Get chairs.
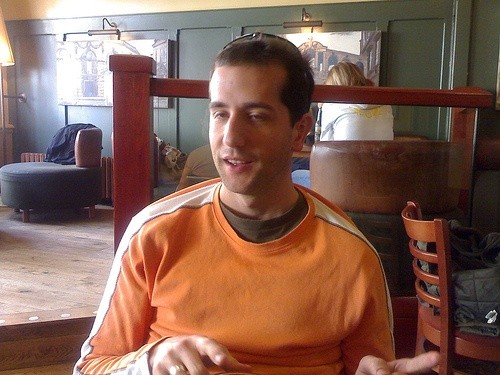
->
[401,200,500,375]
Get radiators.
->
[21,152,114,202]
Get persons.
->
[71,30,441,375]
[176,62,395,188]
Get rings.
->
[169,365,185,375]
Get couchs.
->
[0,127,103,223]
[309,139,451,290]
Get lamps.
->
[87,17,121,36]
[282,7,324,29]
[0,7,27,165]
